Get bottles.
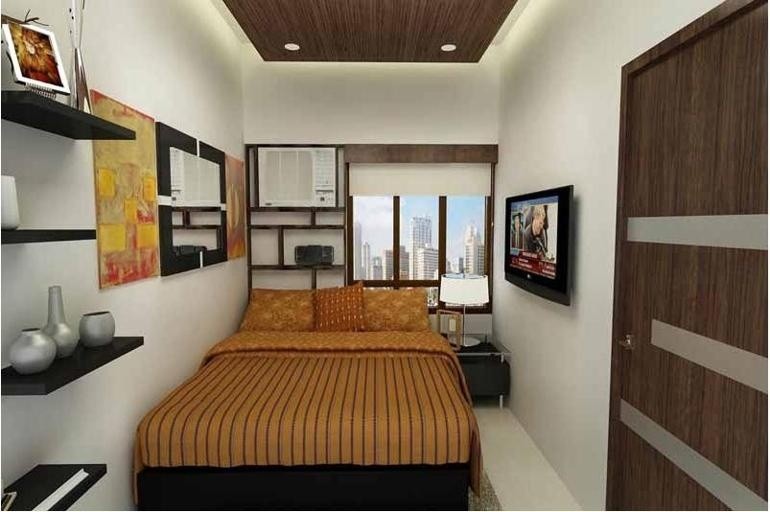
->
[9,287,115,375]
[2,174,20,228]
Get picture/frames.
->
[2,13,72,97]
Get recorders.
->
[294,244,335,265]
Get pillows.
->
[239,289,431,334]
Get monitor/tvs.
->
[503,185,574,308]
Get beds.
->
[132,287,484,511]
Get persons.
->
[511,204,547,261]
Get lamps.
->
[438,272,490,348]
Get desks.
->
[441,333,510,410]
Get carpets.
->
[469,467,502,512]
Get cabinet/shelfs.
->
[1,89,149,511]
[245,145,354,289]
